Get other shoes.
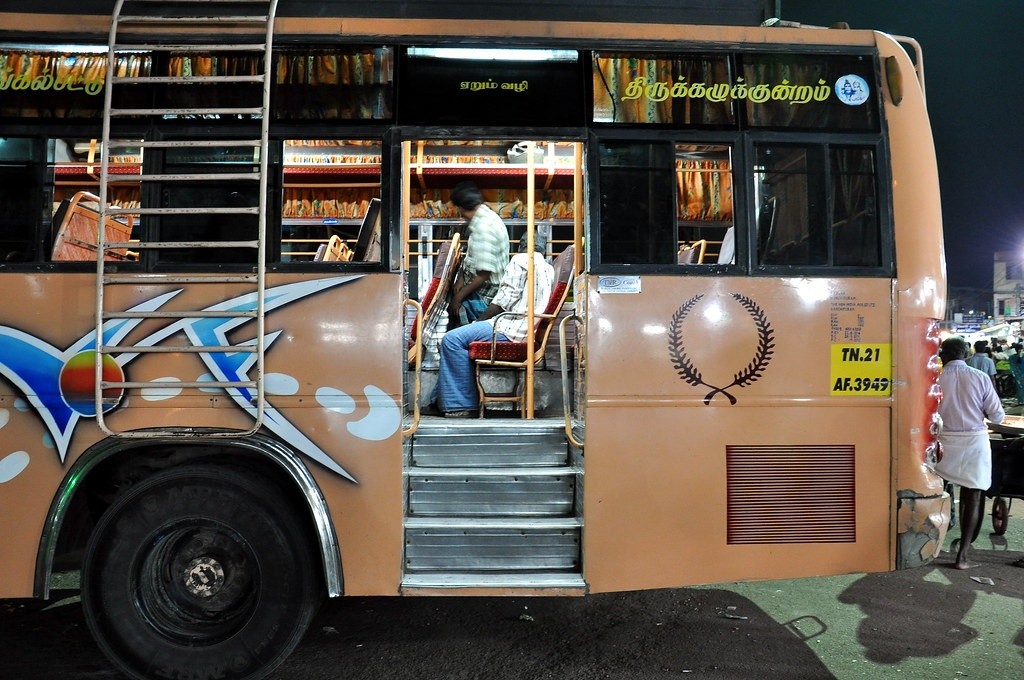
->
[445,405,487,417]
[421,404,443,415]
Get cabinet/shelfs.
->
[51,146,732,226]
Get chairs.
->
[406,233,461,365]
[678,239,707,264]
[314,197,383,262]
[52,189,134,263]
[467,243,577,419]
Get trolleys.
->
[988,435,1024,535]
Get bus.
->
[0,7,952,679]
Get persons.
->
[936,338,1005,571]
[961,329,1024,405]
[448,182,510,326]
[419,231,553,418]
